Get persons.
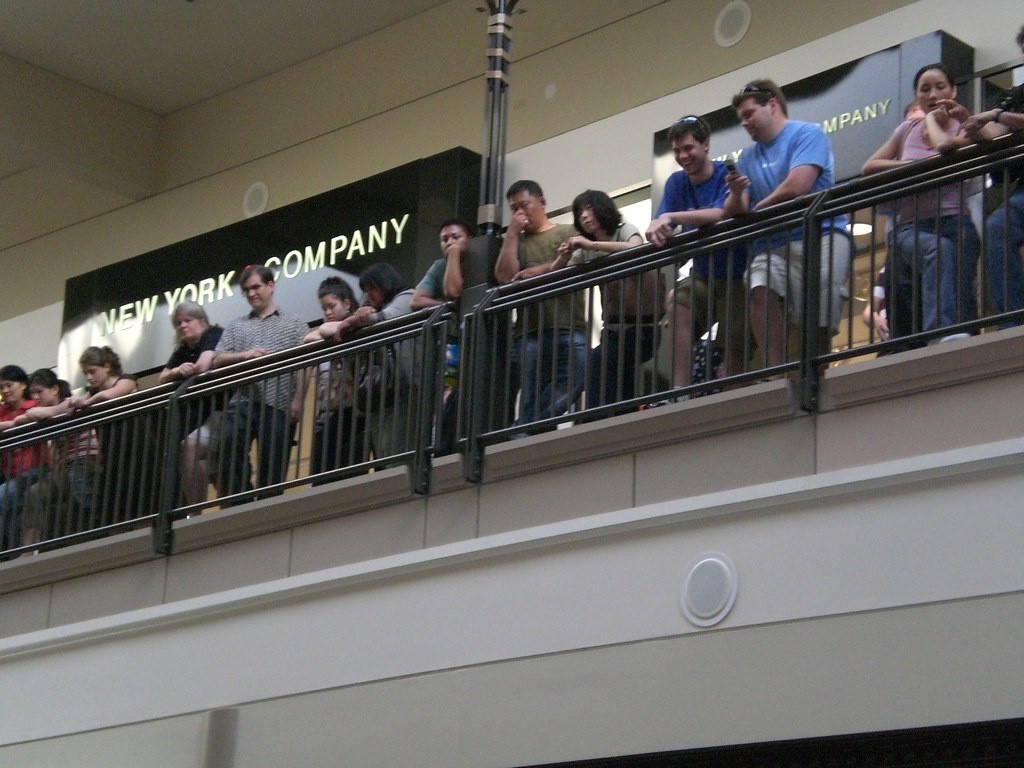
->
[336,263,415,472]
[159,301,225,518]
[550,190,663,424]
[66,346,138,538]
[410,219,491,459]
[862,24,1024,357]
[494,180,588,440]
[0,365,100,563]
[645,115,744,407]
[304,277,360,487]
[212,265,315,506]
[723,80,851,386]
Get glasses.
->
[673,117,706,130]
[740,86,775,96]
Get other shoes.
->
[926,332,973,346]
[640,395,689,410]
[507,424,527,438]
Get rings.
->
[965,123,975,131]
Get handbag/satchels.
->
[359,347,405,401]
[330,358,358,407]
[689,339,723,398]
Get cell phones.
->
[723,159,740,177]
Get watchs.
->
[995,107,1006,123]
